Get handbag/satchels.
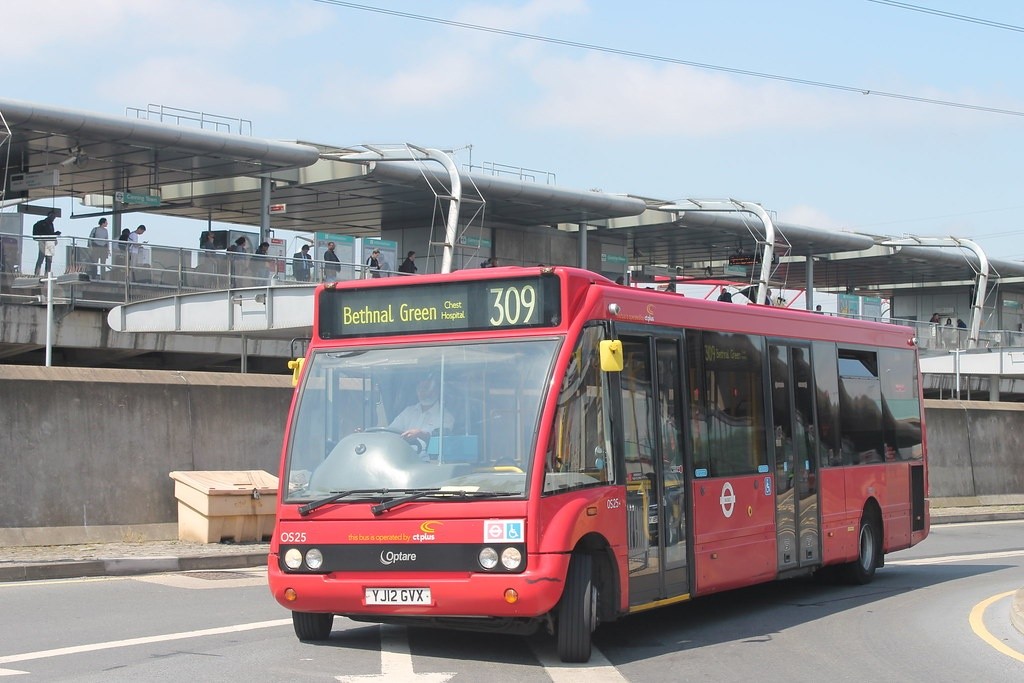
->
[249,258,257,273]
[87,226,97,247]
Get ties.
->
[303,254,307,269]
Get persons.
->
[324,242,341,280]
[383,377,453,465]
[366,250,383,278]
[35,211,61,276]
[718,288,731,302]
[255,242,268,277]
[930,313,940,325]
[488,258,498,267]
[957,319,968,348]
[666,284,675,292]
[128,225,148,282]
[765,288,774,306]
[816,305,821,311]
[616,276,624,285]
[398,251,417,276]
[234,237,246,260]
[944,319,953,347]
[206,232,222,266]
[293,244,313,282]
[88,218,111,279]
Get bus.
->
[267,265,930,662]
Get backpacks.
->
[33,220,44,238]
[118,232,134,250]
[398,260,406,271]
[226,245,237,255]
